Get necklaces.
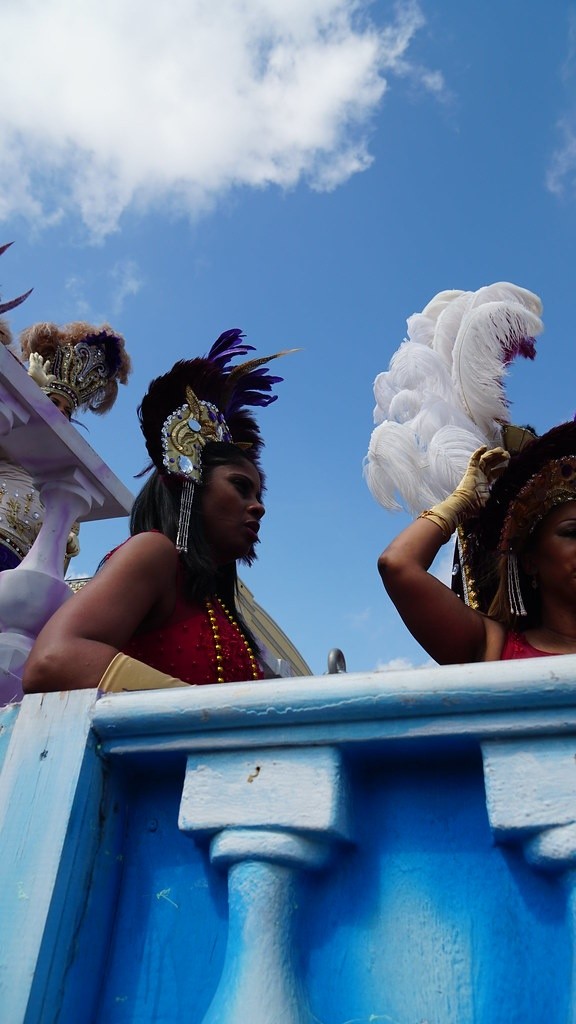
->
[204,592,259,684]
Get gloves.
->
[97,650,191,695]
[418,445,510,546]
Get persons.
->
[364,281,576,666]
[17,320,130,422]
[22,327,301,691]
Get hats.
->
[19,319,130,416]
[135,328,304,560]
[451,416,576,633]
